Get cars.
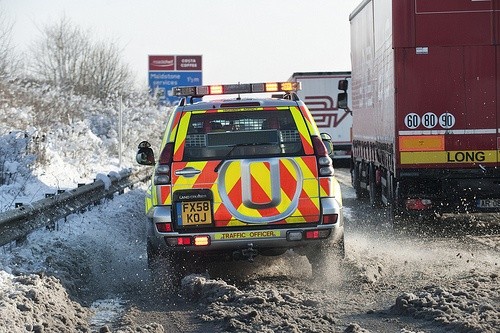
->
[137,82,346,273]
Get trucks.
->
[289,72,352,168]
[349,1,497,229]
